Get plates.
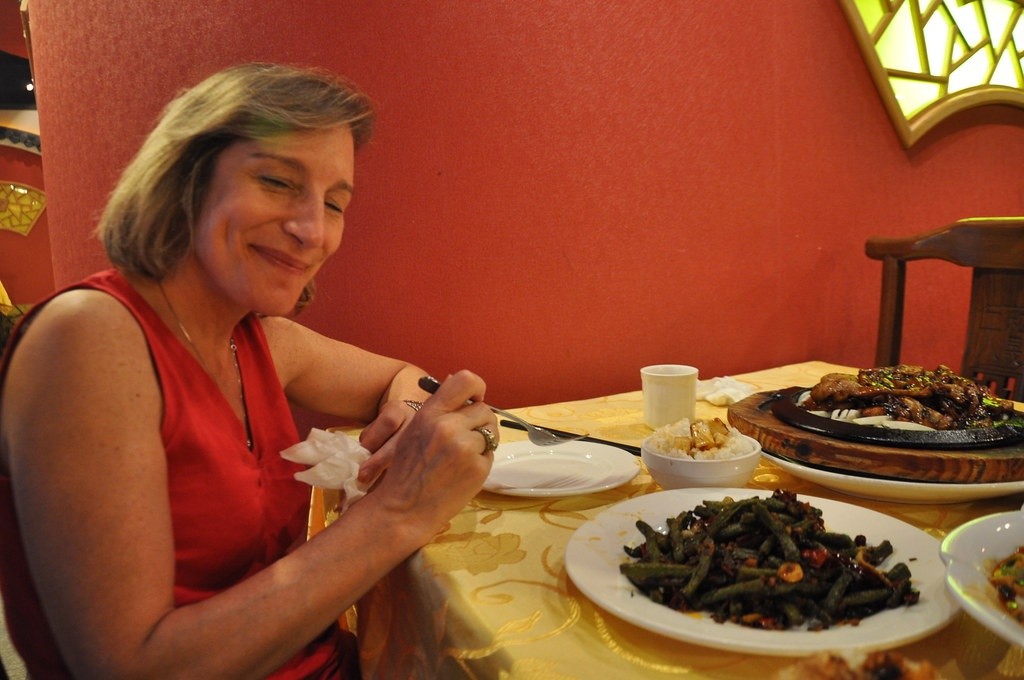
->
[563,485,964,657]
[758,449,1024,505]
[482,440,642,499]
[771,383,1024,450]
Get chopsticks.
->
[499,418,642,456]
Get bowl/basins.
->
[939,506,1024,651]
[641,434,763,491]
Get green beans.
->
[620,489,922,629]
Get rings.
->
[476,426,498,454]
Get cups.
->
[639,364,699,432]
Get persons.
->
[0,60,498,680]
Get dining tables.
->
[301,358,1024,680]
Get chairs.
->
[864,215,1024,403]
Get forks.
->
[418,375,591,448]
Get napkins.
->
[696,374,760,409]
[278,427,381,511]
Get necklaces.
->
[155,277,251,447]
